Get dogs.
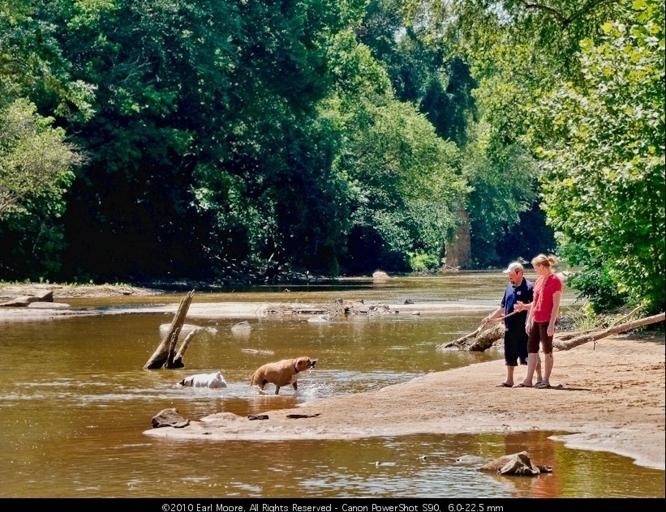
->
[250,356,317,395]
[179,370,227,388]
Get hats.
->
[502,262,524,275]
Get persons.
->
[480,262,545,387]
[512,253,562,389]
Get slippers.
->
[494,381,552,389]
